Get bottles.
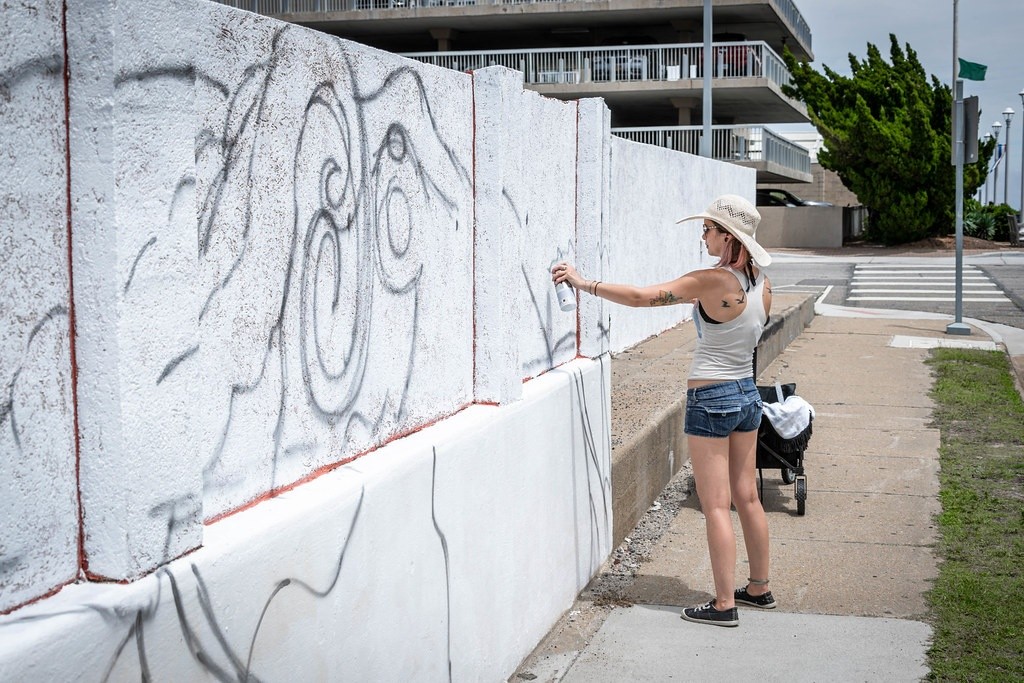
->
[553,266,577,312]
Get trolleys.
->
[754,384,807,514]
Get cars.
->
[757,188,835,207]
[1018,224,1024,243]
[592,50,667,77]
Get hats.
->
[677,194,772,267]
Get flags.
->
[990,144,1006,173]
[958,58,987,81]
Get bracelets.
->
[595,282,602,296]
[590,280,596,295]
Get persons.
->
[551,195,777,626]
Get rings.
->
[563,266,566,270]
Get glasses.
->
[702,224,720,233]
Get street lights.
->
[1020,91,1024,224]
[991,122,1002,202]
[984,133,991,204]
[1002,108,1015,204]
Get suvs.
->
[697,32,761,77]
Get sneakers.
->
[734,584,777,608]
[682,598,739,626]
